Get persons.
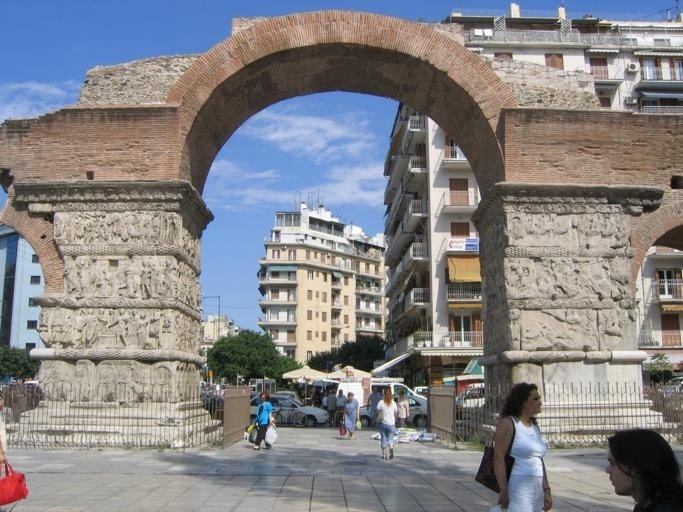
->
[309,386,347,427]
[366,386,383,427]
[490,383,553,512]
[342,393,360,441]
[0,400,7,465]
[396,390,410,428]
[605,428,683,512]
[251,392,275,450]
[373,387,398,460]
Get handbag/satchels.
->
[356,421,362,430]
[249,426,260,443]
[0,473,28,507]
[339,425,347,436]
[475,445,515,494]
[265,428,278,445]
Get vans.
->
[457,382,485,408]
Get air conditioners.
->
[626,62,639,73]
[625,96,637,105]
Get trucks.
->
[337,381,429,409]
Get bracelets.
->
[542,486,550,491]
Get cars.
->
[657,377,682,403]
[201,381,329,427]
[360,394,428,426]
[413,384,428,395]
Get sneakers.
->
[383,448,394,460]
[253,445,272,450]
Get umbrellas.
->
[327,365,372,393]
[282,365,327,401]
